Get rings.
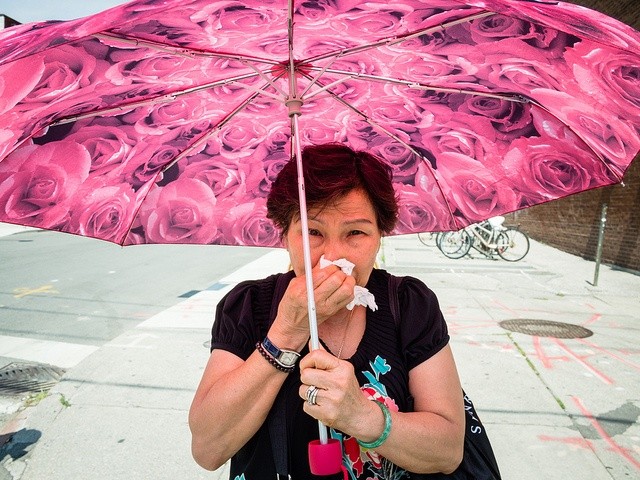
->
[305,385,320,405]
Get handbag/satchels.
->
[387,276,502,480]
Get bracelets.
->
[357,400,392,449]
[256,341,293,373]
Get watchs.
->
[263,337,301,368]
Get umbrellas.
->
[0,0,640,475]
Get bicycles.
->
[417,216,529,262]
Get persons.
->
[189,143,466,480]
